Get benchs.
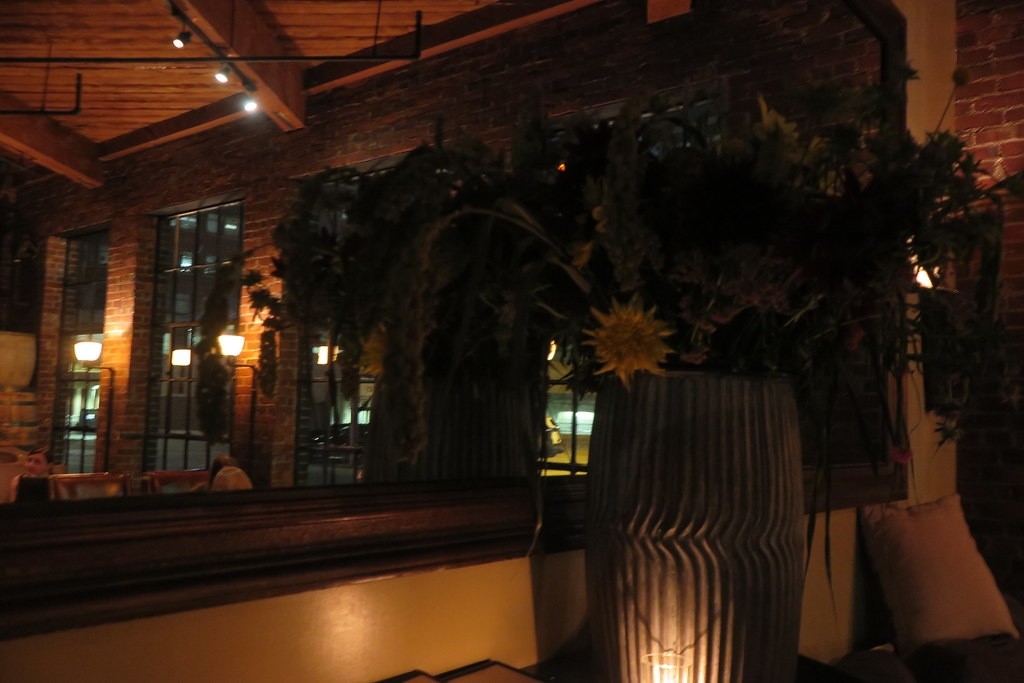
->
[142,468,215,495]
[12,472,133,500]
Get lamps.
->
[214,66,231,84]
[172,22,192,49]
[216,334,255,369]
[73,341,115,373]
[240,97,257,112]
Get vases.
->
[583,365,806,683]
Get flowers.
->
[235,62,1024,623]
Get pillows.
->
[855,493,1020,658]
